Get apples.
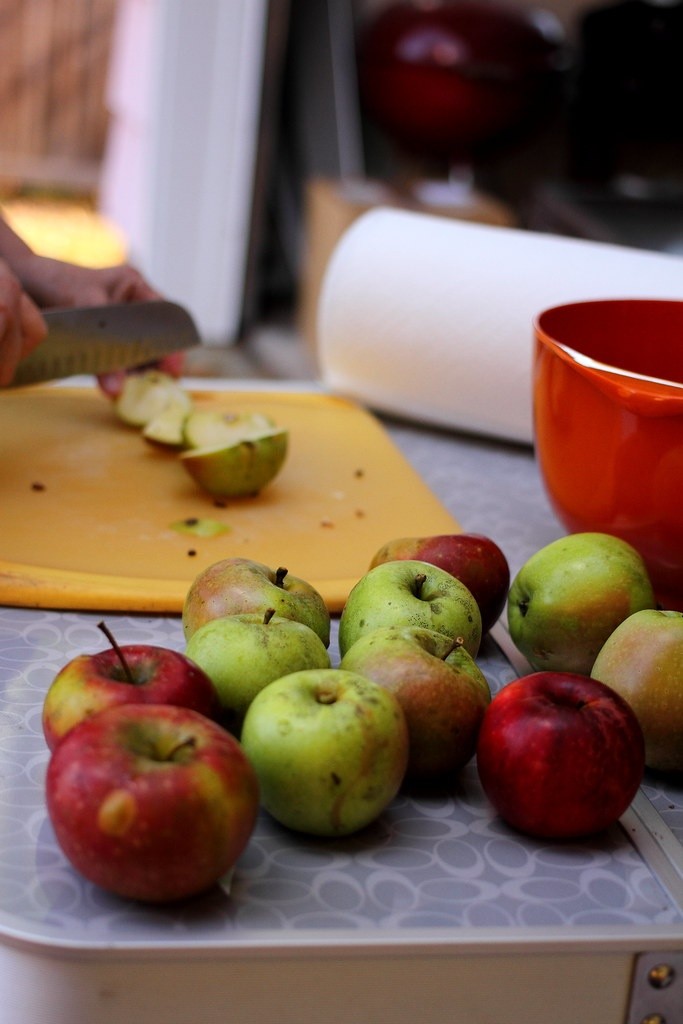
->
[40,531,683,904]
[113,369,287,495]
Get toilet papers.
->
[315,204,683,447]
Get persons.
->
[0,219,186,401]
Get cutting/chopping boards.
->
[0,377,464,618]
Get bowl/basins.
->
[535,299,683,584]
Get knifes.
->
[1,300,202,390]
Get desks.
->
[0,376,683,1024]
